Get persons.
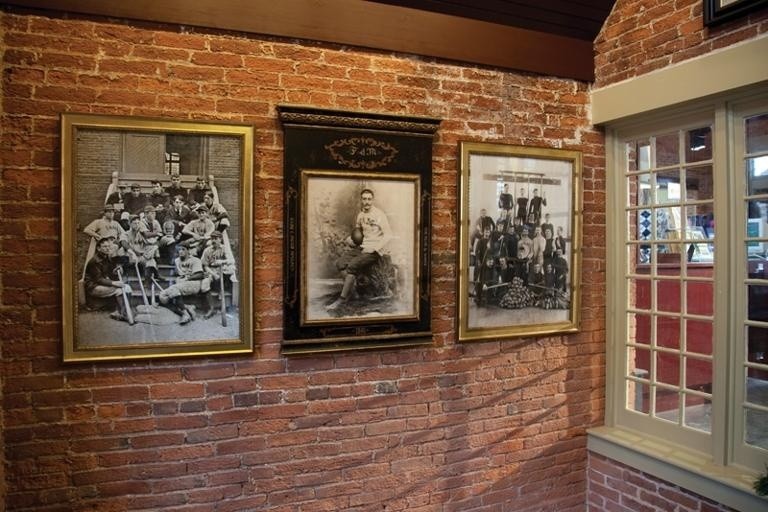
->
[83,174,230,326]
[326,189,392,310]
[470,184,569,308]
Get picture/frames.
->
[279,106,443,358]
[456,138,583,343]
[59,111,255,362]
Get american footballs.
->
[351,227,364,246]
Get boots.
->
[111,293,132,320]
[202,288,217,319]
[159,295,197,325]
[215,278,222,301]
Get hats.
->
[104,180,222,249]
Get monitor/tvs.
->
[747,218,768,257]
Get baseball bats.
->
[151,272,156,304]
[135,264,150,305]
[151,276,163,291]
[220,264,227,326]
[116,268,135,324]
